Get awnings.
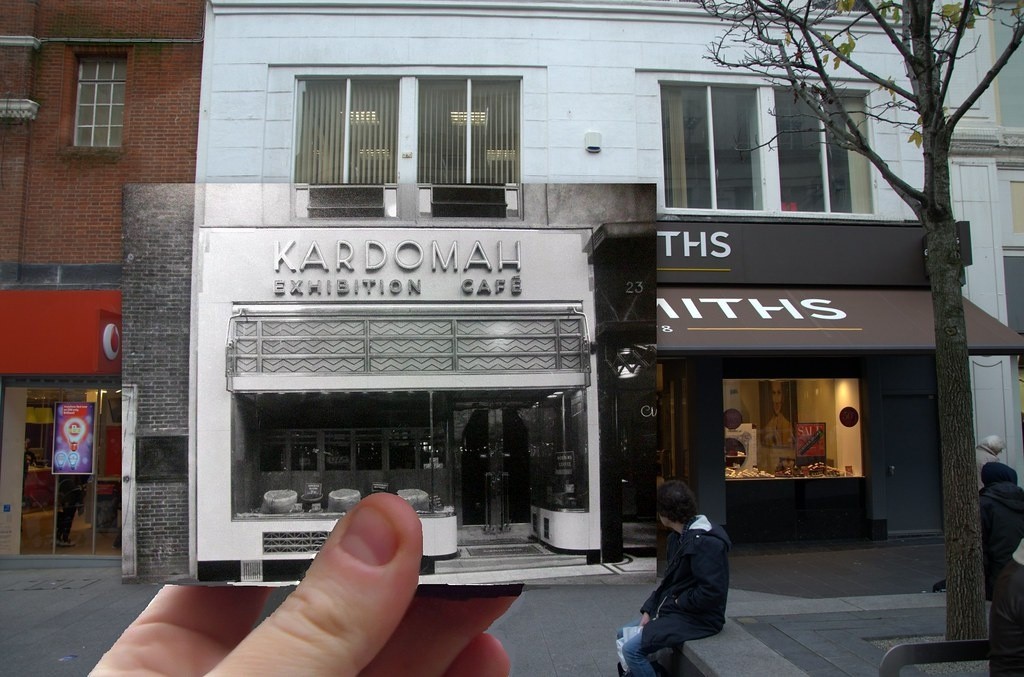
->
[225,304,593,395]
[656,288,1024,363]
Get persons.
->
[85,491,527,677]
[617,478,733,677]
[763,380,793,446]
[987,539,1024,677]
[54,475,91,548]
[977,462,1023,599]
[22,438,39,509]
[974,433,1006,489]
[112,484,122,550]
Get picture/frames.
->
[759,381,799,449]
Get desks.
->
[97,477,123,485]
[25,466,56,509]
